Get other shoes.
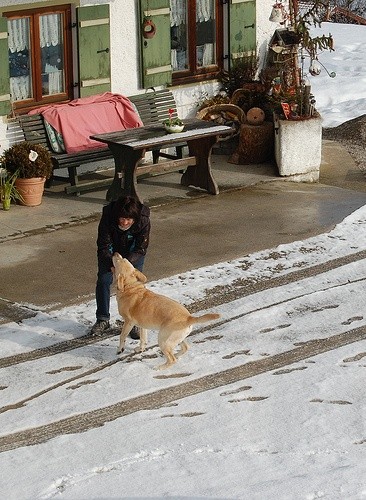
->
[91,319,110,337]
[130,326,141,339]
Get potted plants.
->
[201,44,277,165]
[3,141,54,207]
[0,167,26,210]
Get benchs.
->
[17,88,196,197]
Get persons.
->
[88,196,151,339]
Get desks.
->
[89,118,235,205]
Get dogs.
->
[111,252,222,371]
